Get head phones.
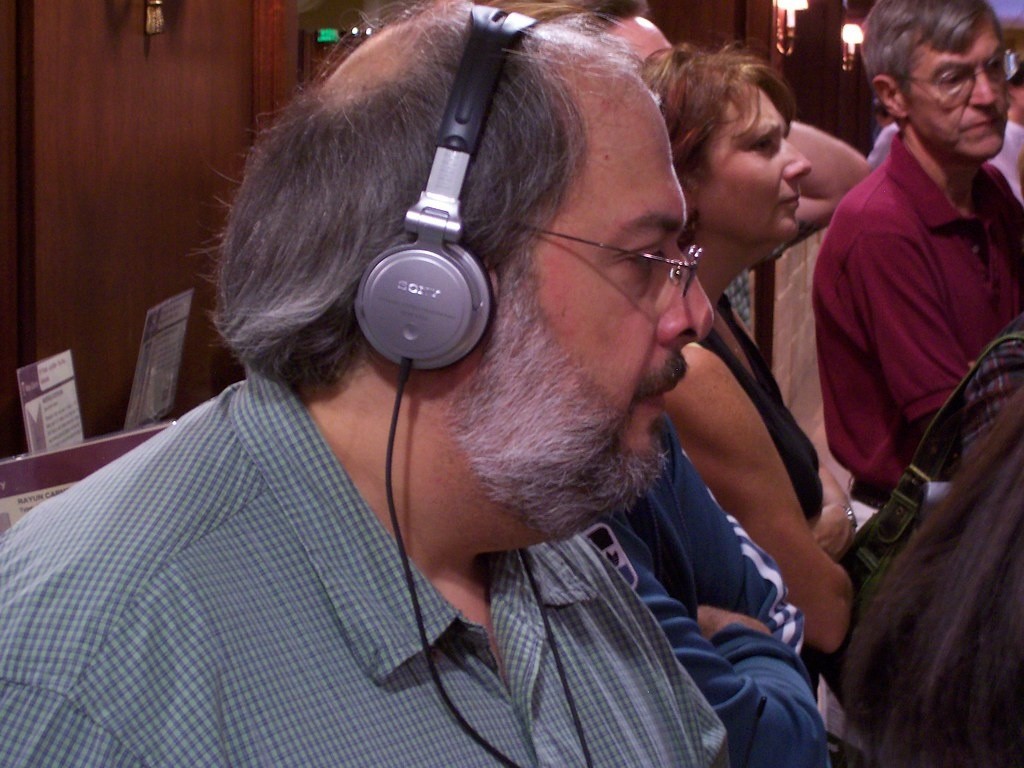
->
[352,3,539,371]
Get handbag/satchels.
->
[819,331,1024,709]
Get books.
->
[16,349,85,455]
[123,288,193,433]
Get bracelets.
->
[836,501,855,522]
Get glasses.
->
[902,48,1019,110]
[513,221,705,298]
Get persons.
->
[0,1,732,768]
[515,2,1024,768]
